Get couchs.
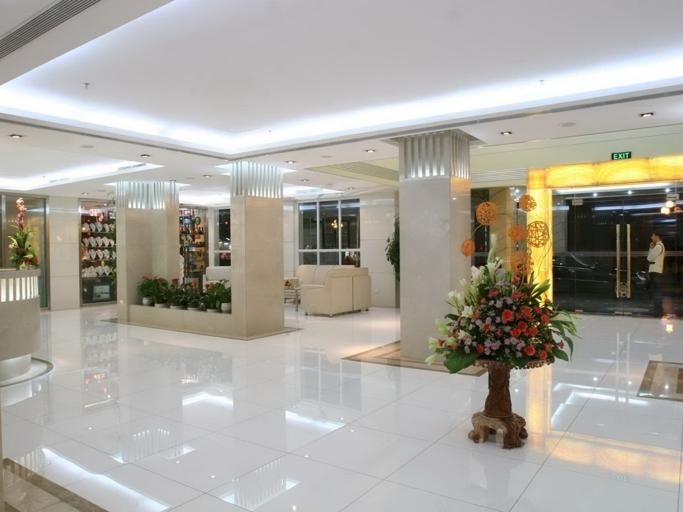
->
[295,264,371,318]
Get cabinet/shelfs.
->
[180,216,209,276]
[82,214,117,302]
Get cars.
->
[554,252,650,296]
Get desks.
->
[464,357,556,452]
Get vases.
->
[142,297,231,313]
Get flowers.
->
[425,255,579,377]
[137,275,231,306]
[7,196,40,269]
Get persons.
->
[644,228,665,318]
